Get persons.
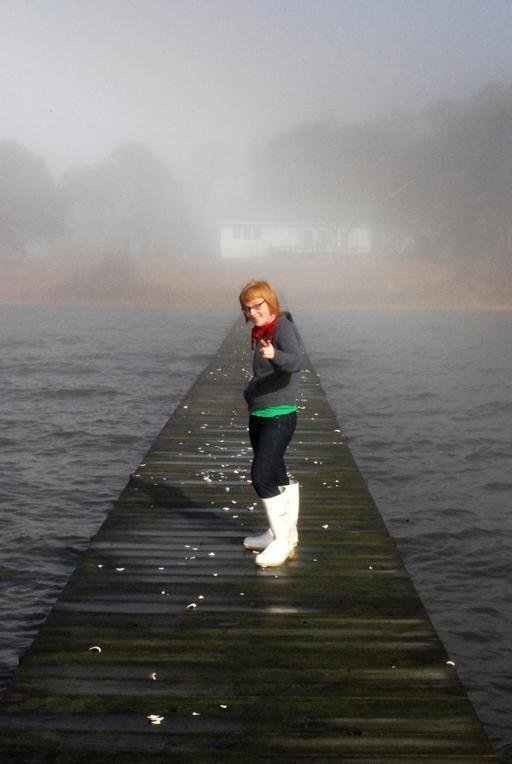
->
[238,280,307,570]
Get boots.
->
[255,487,296,567]
[243,479,300,551]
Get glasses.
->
[241,301,267,315]
